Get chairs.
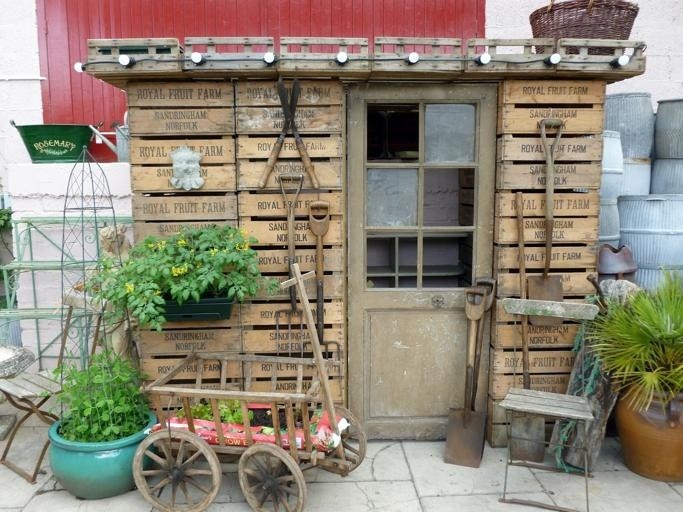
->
[498,298,602,512]
[0,293,107,484]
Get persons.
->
[86,222,137,376]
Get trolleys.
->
[131,261,366,512]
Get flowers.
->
[100,224,284,333]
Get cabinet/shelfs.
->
[367,232,465,288]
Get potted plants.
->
[39,350,157,499]
[584,269,683,482]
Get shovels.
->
[471,277,497,462]
[443,286,489,468]
[510,191,558,463]
[527,119,564,328]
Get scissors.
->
[257,75,321,190]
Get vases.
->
[155,295,234,323]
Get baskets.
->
[528,0,640,56]
